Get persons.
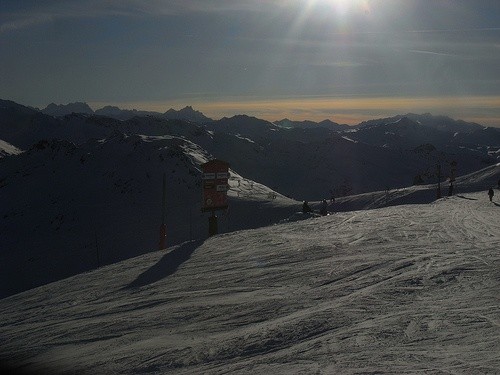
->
[303,200,313,214]
[159,223,166,249]
[487,187,495,201]
